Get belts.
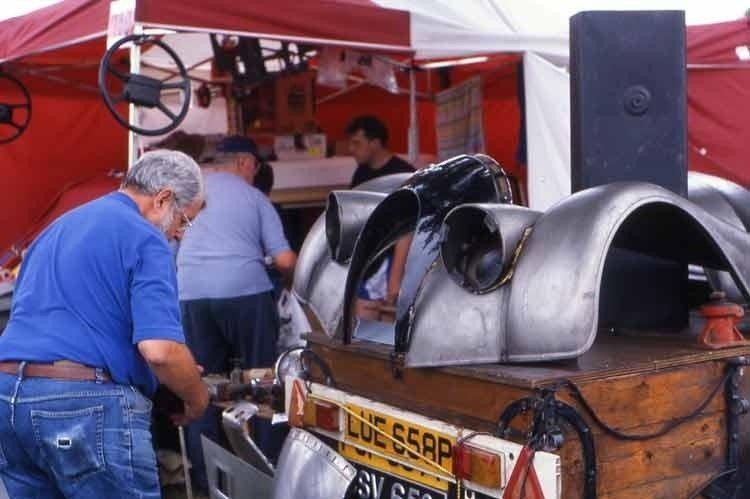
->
[0,361,109,382]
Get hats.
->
[216,135,256,152]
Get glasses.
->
[175,195,193,231]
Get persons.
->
[176,135,296,499]
[0,148,211,499]
[254,167,291,241]
[345,115,416,188]
